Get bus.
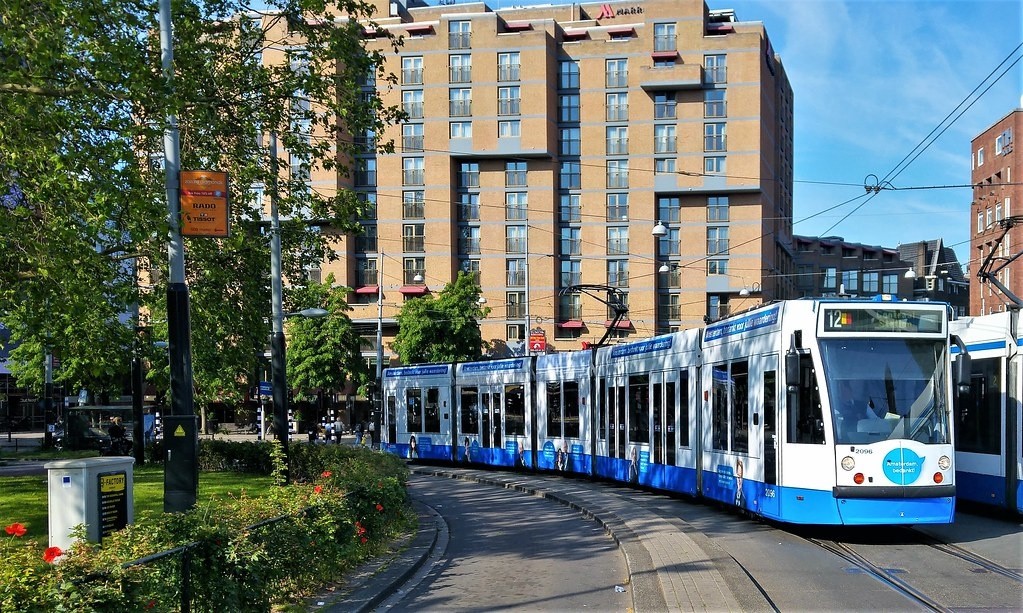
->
[382,283,973,527]
[67,405,155,447]
[944,215,1023,515]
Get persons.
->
[629,447,639,484]
[735,461,747,509]
[515,441,529,467]
[555,448,565,471]
[355,421,365,448]
[334,417,343,445]
[463,438,472,462]
[407,438,418,458]
[308,420,319,444]
[367,418,375,448]
[563,441,573,471]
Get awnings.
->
[405,24,433,34]
[651,51,678,59]
[399,286,427,293]
[607,28,635,37]
[705,26,733,31]
[604,320,631,329]
[504,23,531,29]
[562,320,585,328]
[354,286,379,294]
[365,29,377,34]
[563,31,588,37]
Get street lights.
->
[130,252,168,467]
[268,127,329,486]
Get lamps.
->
[659,262,670,273]
[651,222,669,237]
[478,297,488,303]
[905,268,916,280]
[412,273,424,282]
[739,288,751,298]
